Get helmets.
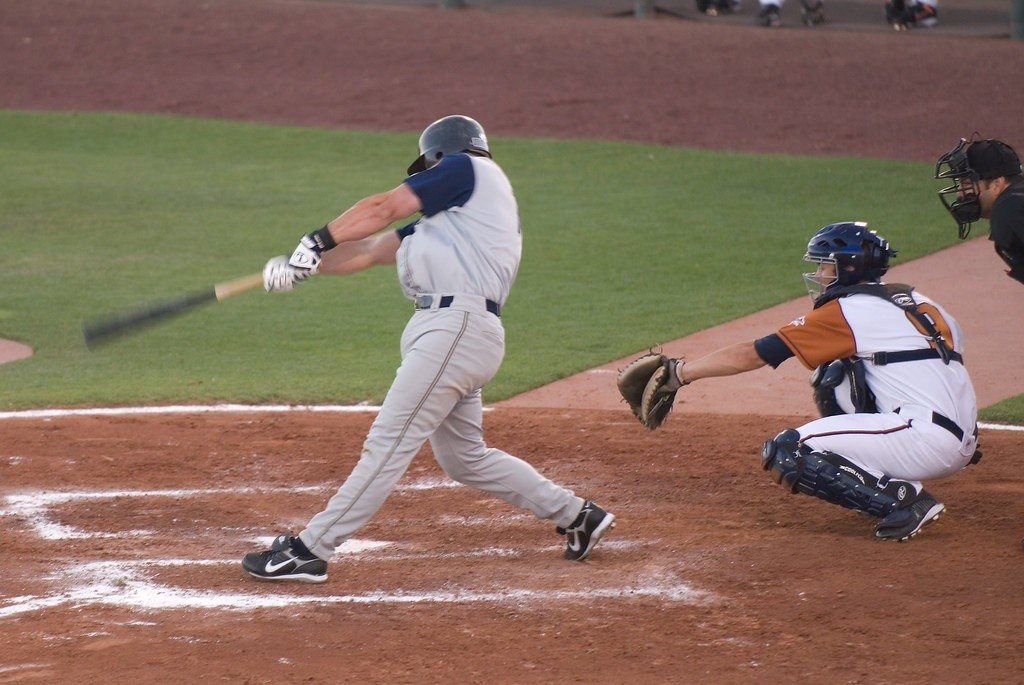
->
[948,138,1022,179]
[802,222,898,266]
[407,114,491,175]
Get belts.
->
[893,406,964,441]
[416,295,500,316]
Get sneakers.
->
[554,499,615,561]
[242,531,329,584]
[875,489,947,542]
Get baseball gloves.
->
[616,341,687,430]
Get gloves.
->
[263,254,322,293]
[288,223,337,291]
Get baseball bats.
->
[82,264,320,351]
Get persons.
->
[241,114,617,587]
[618,222,982,540]
[696,0,937,32]
[935,131,1024,288]
